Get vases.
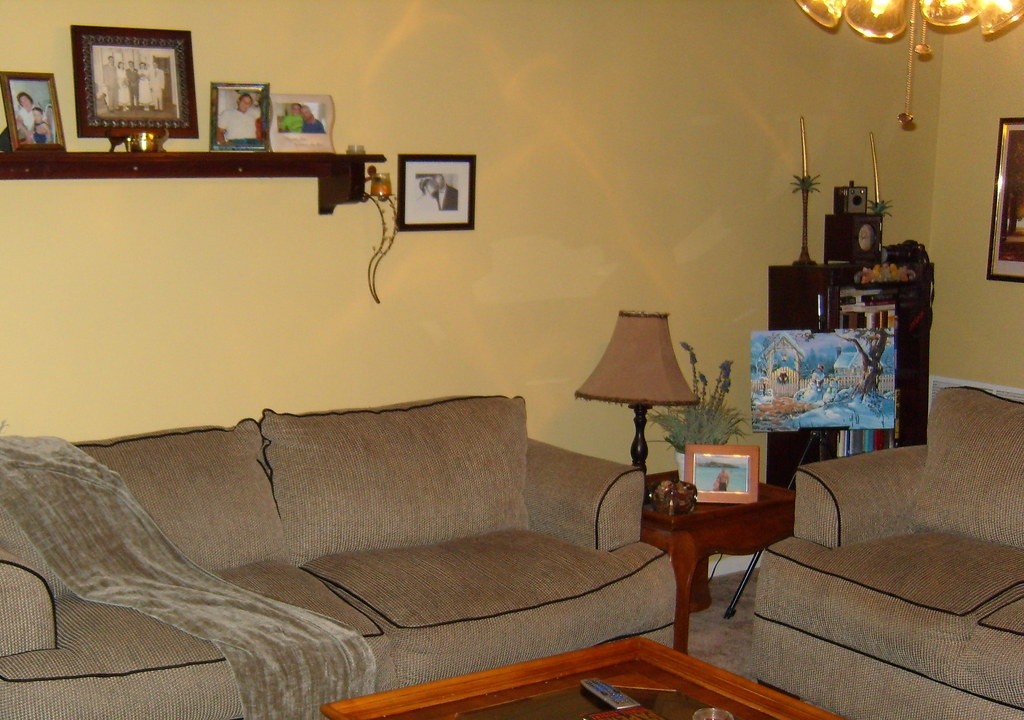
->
[674,452,687,481]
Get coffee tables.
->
[319,635,846,720]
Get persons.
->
[280,102,324,134]
[416,174,457,212]
[716,468,729,491]
[103,56,165,112]
[17,91,52,144]
[217,92,261,146]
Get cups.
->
[131,132,158,152]
[371,173,391,196]
[692,708,734,720]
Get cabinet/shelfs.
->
[765,265,938,487]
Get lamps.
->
[578,311,698,477]
[794,1,1021,132]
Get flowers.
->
[649,343,743,453]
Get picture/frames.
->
[270,95,336,151]
[69,25,200,135]
[1,72,67,149]
[206,83,270,151]
[396,153,478,230]
[987,117,1024,283]
[687,445,762,505]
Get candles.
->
[798,114,808,177]
[869,131,883,204]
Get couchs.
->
[1,397,675,720]
[751,387,1023,719]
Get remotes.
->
[580,676,641,710]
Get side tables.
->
[635,471,802,661]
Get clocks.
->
[825,213,883,264]
